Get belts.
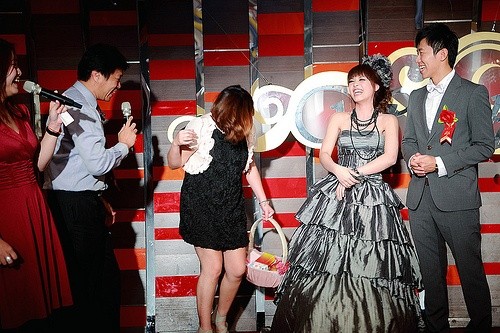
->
[425,178,429,186]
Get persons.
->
[400,23,494,333]
[167,85,275,333]
[38,44,138,333]
[269,52,428,333]
[0,35,75,333]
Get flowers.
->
[276,262,291,275]
[437,104,458,144]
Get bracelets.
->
[260,200,269,205]
[47,128,63,137]
[434,163,438,172]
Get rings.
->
[5,257,11,263]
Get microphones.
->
[22,81,83,109]
[121,102,133,152]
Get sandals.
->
[198,327,213,333]
[211,304,230,333]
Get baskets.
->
[245,217,288,288]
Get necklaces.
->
[350,107,380,161]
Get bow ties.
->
[426,82,445,93]
